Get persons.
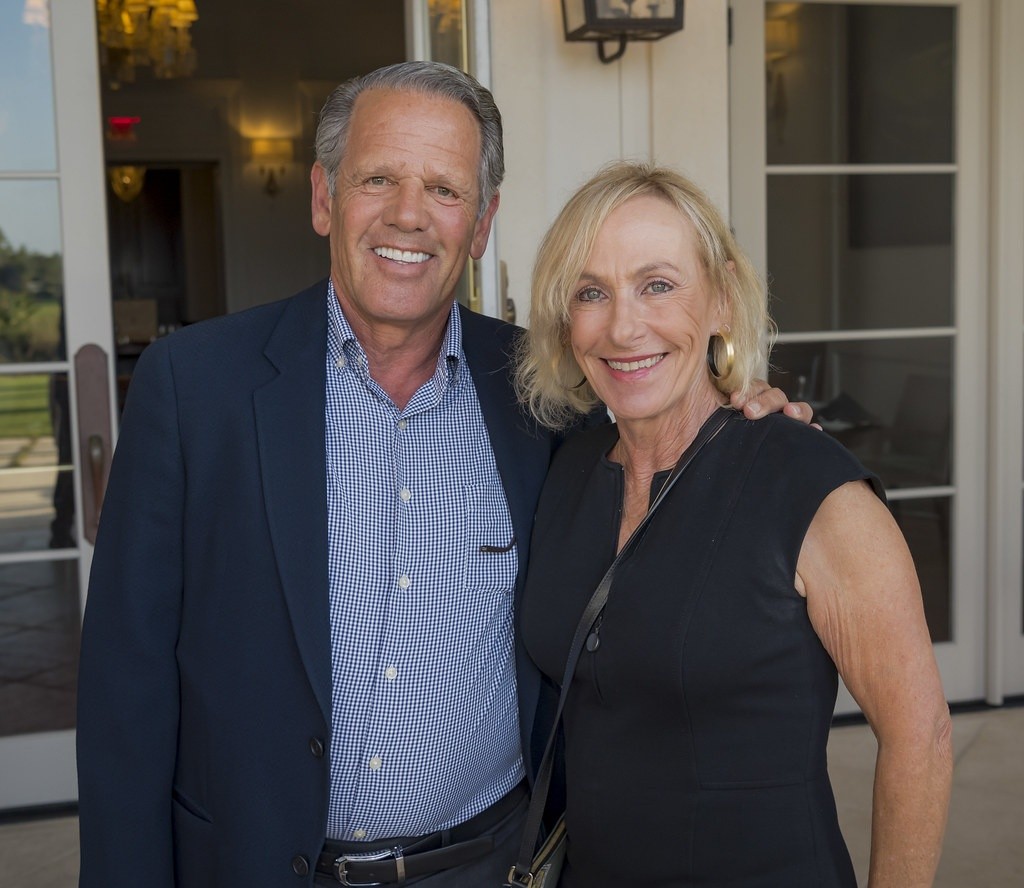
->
[77,60,823,888]
[512,163,955,888]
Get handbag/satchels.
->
[501,812,569,888]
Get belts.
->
[316,776,530,887]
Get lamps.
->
[562,0,684,63]
